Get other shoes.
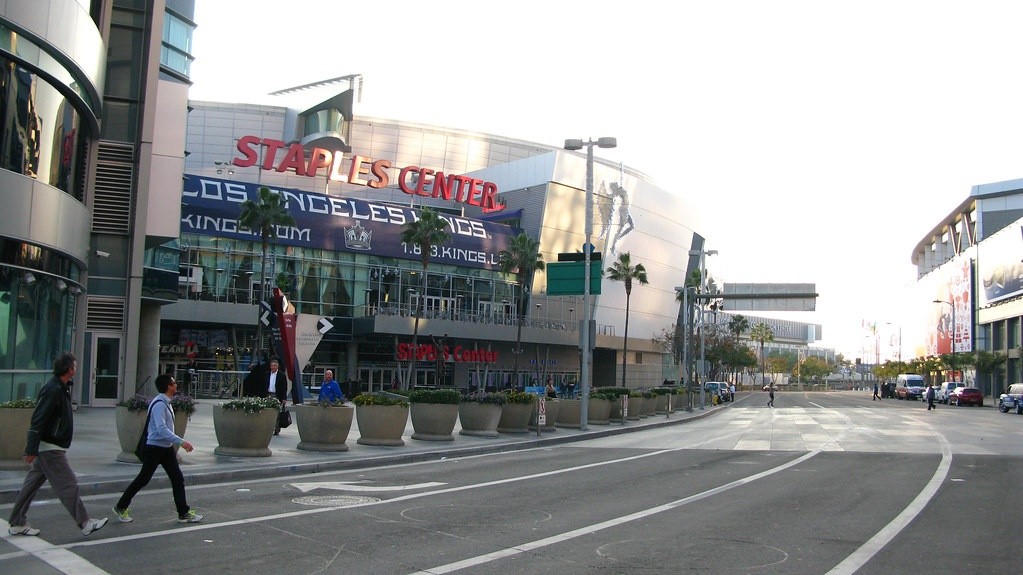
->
[273,432,278,435]
[771,405,774,407]
[767,403,769,406]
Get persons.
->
[392,376,400,390]
[767,382,774,407]
[926,384,936,410]
[8,352,108,536]
[112,374,204,523]
[713,382,735,406]
[545,377,575,398]
[260,359,288,435]
[318,370,344,405]
[873,384,881,401]
[185,359,255,395]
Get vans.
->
[894,372,927,400]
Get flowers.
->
[116,392,199,422]
[218,396,282,416]
[0,397,38,408]
[505,393,537,404]
[409,388,461,404]
[351,393,408,407]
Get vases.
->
[496,404,534,434]
[357,404,409,446]
[212,404,279,457]
[116,405,189,464]
[554,399,581,428]
[0,408,37,470]
[410,402,459,440]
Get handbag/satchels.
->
[134,432,148,461]
[280,403,292,427]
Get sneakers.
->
[81,517,108,535]
[113,505,134,522]
[177,508,204,523]
[9,525,40,536]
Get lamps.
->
[0,291,11,305]
[56,277,67,292]
[70,284,82,297]
[19,272,36,286]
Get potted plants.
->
[597,387,630,422]
[527,397,559,432]
[296,395,354,451]
[639,391,653,418]
[460,391,507,436]
[647,393,658,416]
[670,387,710,411]
[627,390,644,420]
[580,393,616,424]
[653,388,670,414]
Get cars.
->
[937,382,965,404]
[949,387,983,407]
[763,384,778,391]
[705,381,731,402]
[921,385,941,402]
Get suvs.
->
[999,382,1023,415]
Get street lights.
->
[564,135,619,433]
[761,337,766,387]
[675,249,720,414]
[866,335,880,366]
[933,300,959,383]
[795,345,803,387]
[886,322,902,374]
